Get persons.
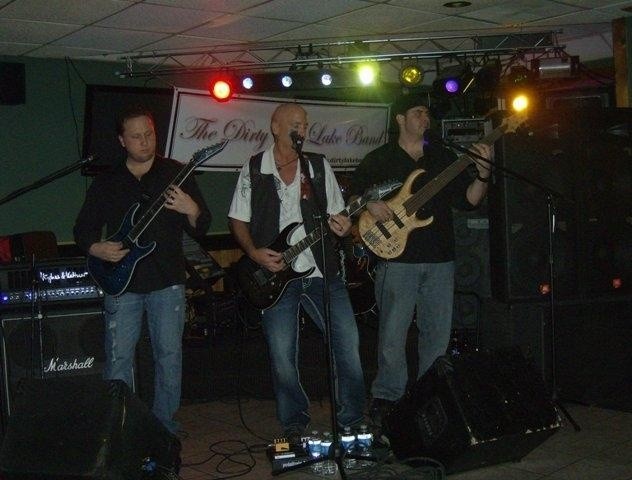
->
[227,102,368,444]
[346,94,493,427]
[73,102,211,435]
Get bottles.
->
[308,424,374,477]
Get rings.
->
[339,227,343,231]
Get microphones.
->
[423,129,449,147]
[289,131,305,154]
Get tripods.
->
[451,144,581,432]
[271,149,392,480]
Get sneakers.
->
[337,420,369,433]
[284,423,305,437]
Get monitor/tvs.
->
[81,84,204,176]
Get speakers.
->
[0,62,26,105]
[487,133,632,302]
[0,378,181,480]
[383,349,565,475]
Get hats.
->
[390,95,427,115]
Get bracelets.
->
[476,173,493,183]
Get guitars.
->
[86,139,228,298]
[227,178,403,313]
[358,108,527,259]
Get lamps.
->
[211,69,234,103]
[432,58,469,98]
[359,57,381,82]
[317,63,335,89]
[512,86,530,112]
[398,58,425,88]
[240,73,257,92]
[281,65,298,89]
